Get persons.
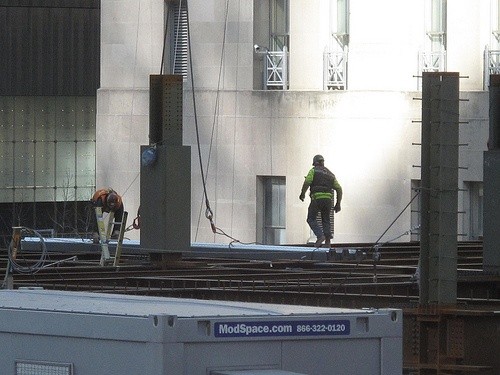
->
[90,188,124,243]
[300,155,342,248]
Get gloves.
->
[300,193,305,201]
[335,203,340,213]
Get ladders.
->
[98,210,128,266]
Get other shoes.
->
[114,230,120,240]
[322,243,332,248]
[315,236,324,246]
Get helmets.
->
[106,193,117,208]
[312,154,325,165]
